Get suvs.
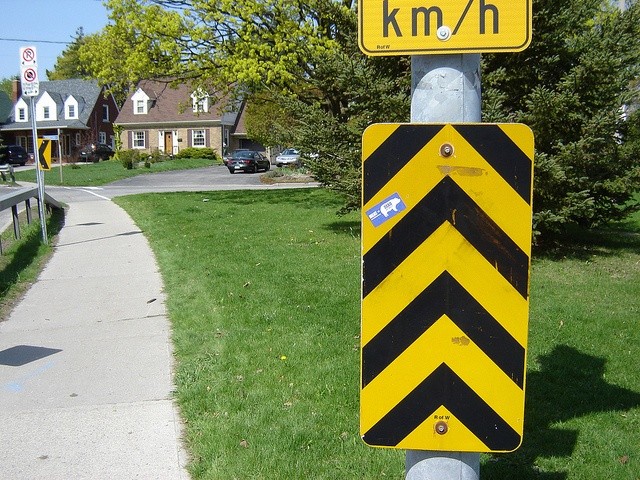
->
[80,143,115,161]
[0,145,29,166]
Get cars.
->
[276,148,300,167]
[226,150,270,174]
[222,148,250,165]
